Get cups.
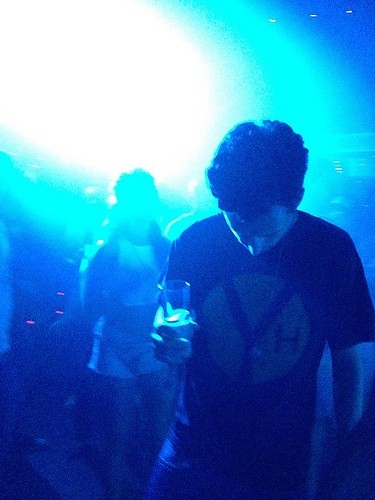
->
[160,279,192,337]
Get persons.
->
[79,169,190,500]
[142,120,374,500]
